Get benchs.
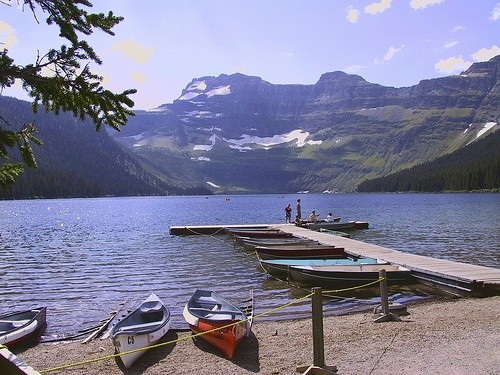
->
[120,321,163,331]
[193,301,221,305]
[190,308,242,320]
[0,319,30,324]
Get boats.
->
[0,306,48,360]
[296,190,309,194]
[291,216,341,224]
[111,292,172,370]
[224,227,412,291]
[182,288,252,361]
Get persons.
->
[284,203,292,223]
[327,213,333,220]
[296,198,301,219]
[309,210,319,223]
[294,215,302,226]
